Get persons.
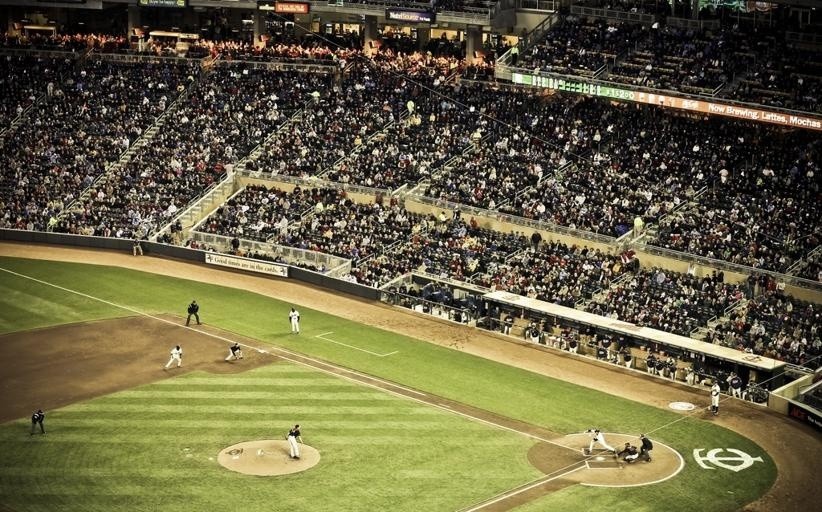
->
[613,442,639,462]
[639,434,653,457]
[30,409,47,435]
[165,345,182,368]
[289,308,300,334]
[0,0,393,288]
[585,429,615,453]
[285,424,303,459]
[710,379,720,415]
[225,343,243,360]
[379,0,822,402]
[185,301,202,326]
